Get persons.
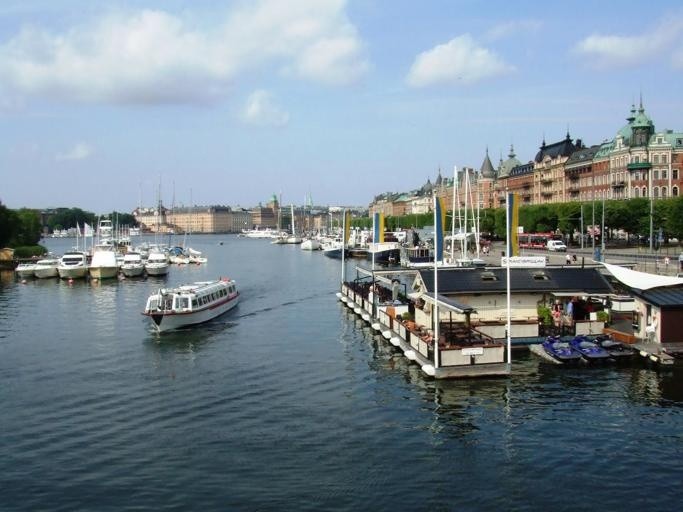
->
[573,253,577,263]
[678,252,683,270]
[486,246,490,256]
[483,245,486,254]
[566,253,570,264]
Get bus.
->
[515,232,563,250]
[477,230,491,248]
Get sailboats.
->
[272,188,314,244]
[52,173,209,267]
[436,162,487,267]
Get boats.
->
[593,330,637,362]
[541,333,582,365]
[138,275,241,335]
[10,251,171,282]
[568,332,612,363]
[237,224,288,240]
[301,227,442,270]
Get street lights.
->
[647,213,668,255]
[327,212,332,231]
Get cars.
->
[546,229,647,252]
